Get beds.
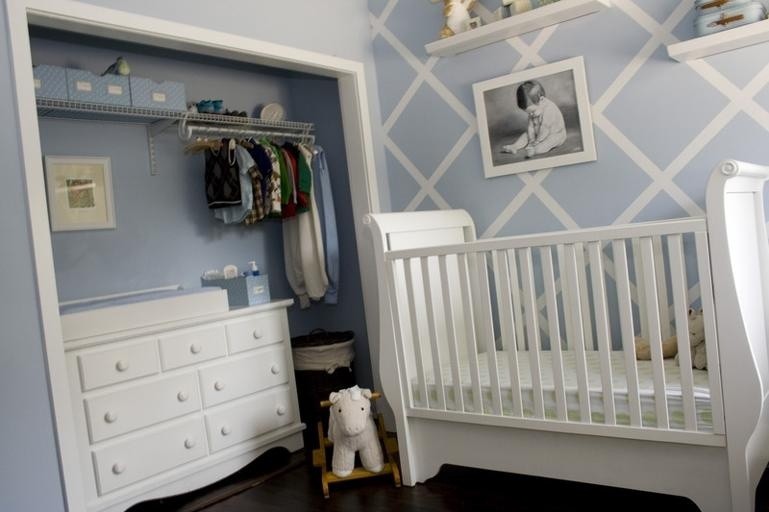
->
[360,159,768,512]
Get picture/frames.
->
[44,154,119,232]
[470,55,598,179]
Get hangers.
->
[179,130,317,155]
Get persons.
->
[499,82,570,156]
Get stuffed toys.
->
[675,307,708,370]
[436,0,479,39]
[312,386,401,495]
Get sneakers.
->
[196,99,224,114]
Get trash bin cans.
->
[290,328,357,437]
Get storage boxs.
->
[32,63,187,124]
